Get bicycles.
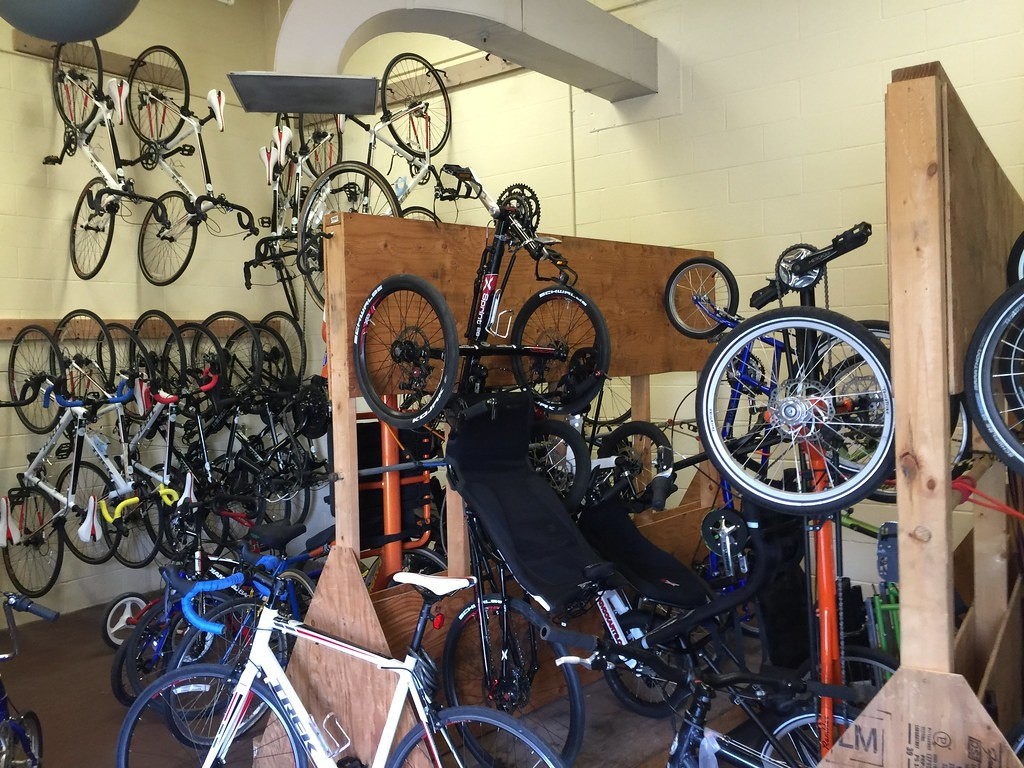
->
[0,38,1024,768]
